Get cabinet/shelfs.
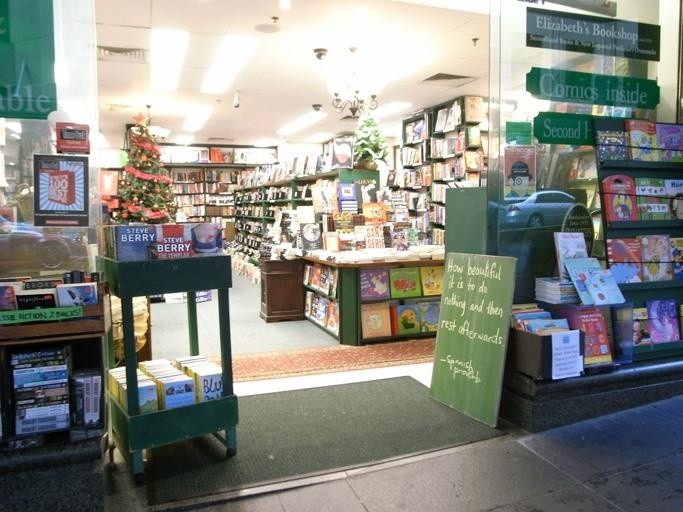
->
[302,247,447,347]
[402,94,516,246]
[163,166,380,346]
[94,250,239,485]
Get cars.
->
[499,190,578,229]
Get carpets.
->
[145,377,508,509]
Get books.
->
[2,120,107,440]
[161,145,231,239]
[504,116,682,379]
[98,223,223,302]
[109,355,223,414]
[231,96,501,340]
[101,169,126,221]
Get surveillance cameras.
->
[312,104,322,111]
[312,49,328,60]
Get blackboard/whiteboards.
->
[431,252,516,428]
